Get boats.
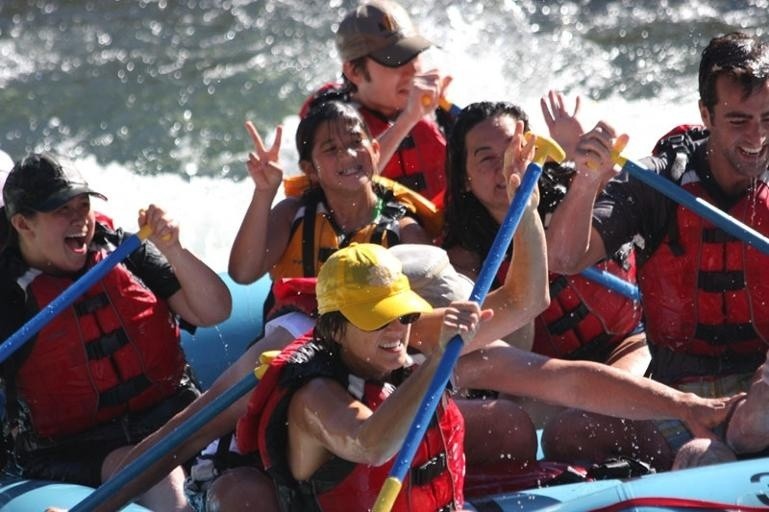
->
[0,262,767,511]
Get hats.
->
[331,0,441,69]
[1,151,109,222]
[385,242,477,311]
[315,241,435,333]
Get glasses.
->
[369,311,422,331]
